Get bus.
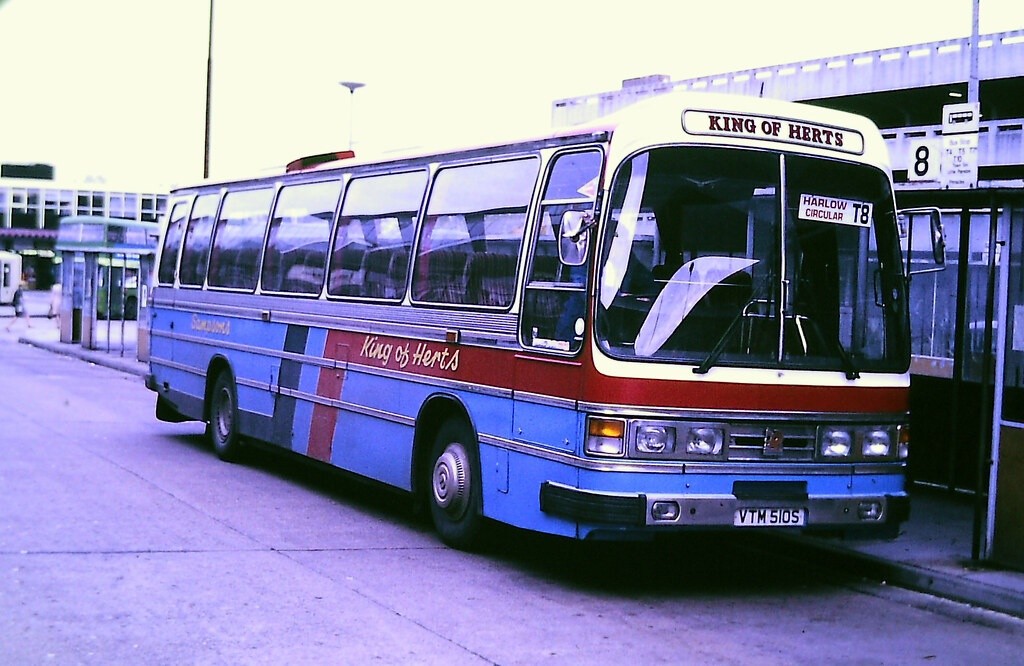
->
[144,92,948,551]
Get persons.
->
[50,280,63,329]
[6,281,33,333]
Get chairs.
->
[196,240,564,338]
